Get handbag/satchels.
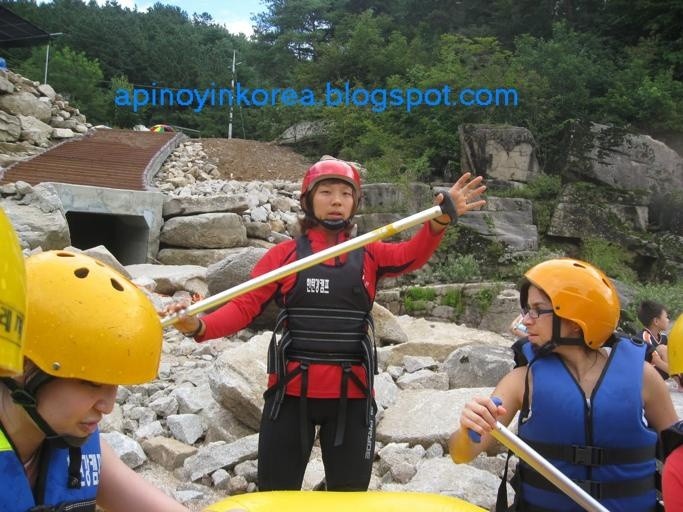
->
[183,320,202,338]
[432,218,449,226]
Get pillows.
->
[0,204,27,379]
[666,313,683,378]
[524,257,621,350]
[23,249,163,385]
[300,160,366,202]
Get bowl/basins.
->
[24,451,37,470]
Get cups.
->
[520,302,553,319]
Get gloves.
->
[201,489,492,512]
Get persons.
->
[0,250,197,512]
[447,257,681,510]
[633,301,683,393]
[156,160,487,490]
